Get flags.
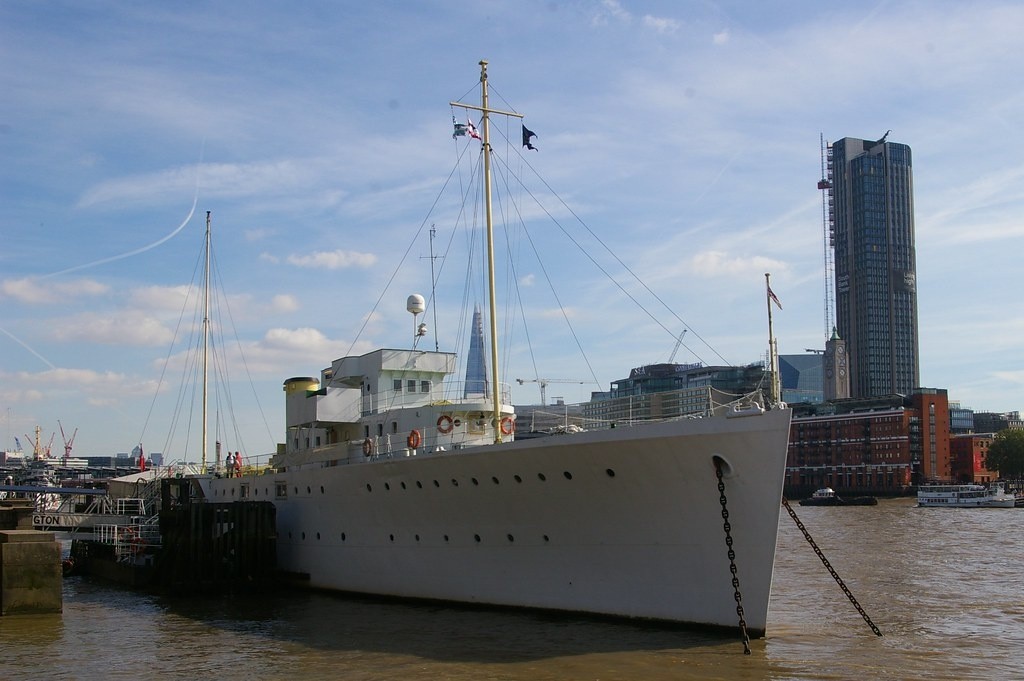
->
[453,115,482,140]
[767,285,782,309]
[139,446,146,471]
[520,123,542,153]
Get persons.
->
[226,452,242,478]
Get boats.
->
[20,460,61,511]
[184,56,792,636]
[916,485,1015,509]
[801,487,877,504]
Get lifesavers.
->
[167,466,173,477]
[501,416,516,434]
[437,415,454,434]
[131,538,145,552]
[363,437,373,458]
[408,429,421,449]
